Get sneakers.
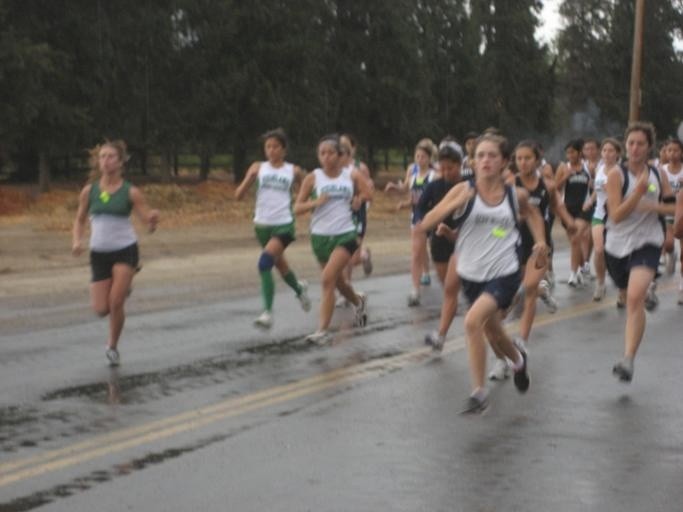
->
[424,334,529,415]
[104,345,119,367]
[419,275,430,285]
[659,251,683,305]
[642,280,659,312]
[254,248,371,345]
[408,291,420,307]
[611,360,634,382]
[513,262,625,318]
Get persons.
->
[72,139,160,365]
[236,126,311,328]
